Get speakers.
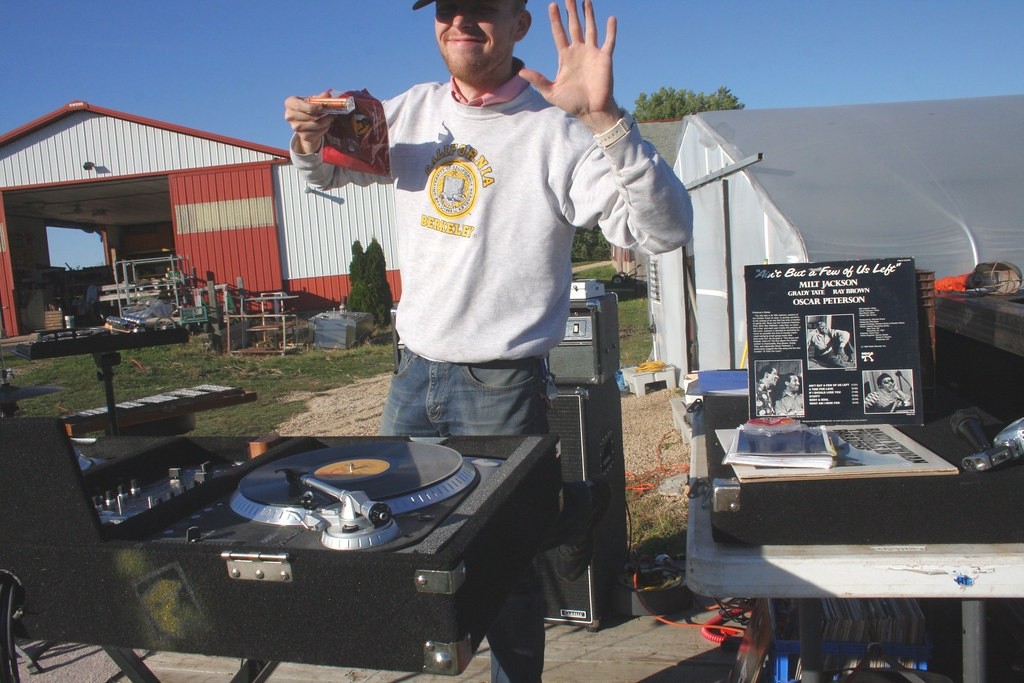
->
[529,377,629,632]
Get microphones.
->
[950,408,992,454]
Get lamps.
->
[83,162,94,170]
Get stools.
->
[621,366,676,396]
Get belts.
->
[463,351,546,370]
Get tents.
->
[612,101,1024,384]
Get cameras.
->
[963,419,1024,472]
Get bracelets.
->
[592,107,633,148]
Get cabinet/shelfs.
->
[114,254,374,356]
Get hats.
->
[412,0,528,10]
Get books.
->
[722,423,838,468]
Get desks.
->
[685,404,1024,683]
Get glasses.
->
[883,379,894,384]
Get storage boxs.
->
[918,271,938,392]
[765,594,932,683]
[570,278,604,300]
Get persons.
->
[755,365,804,415]
[808,321,855,368]
[864,373,911,413]
[284,1,692,683]
